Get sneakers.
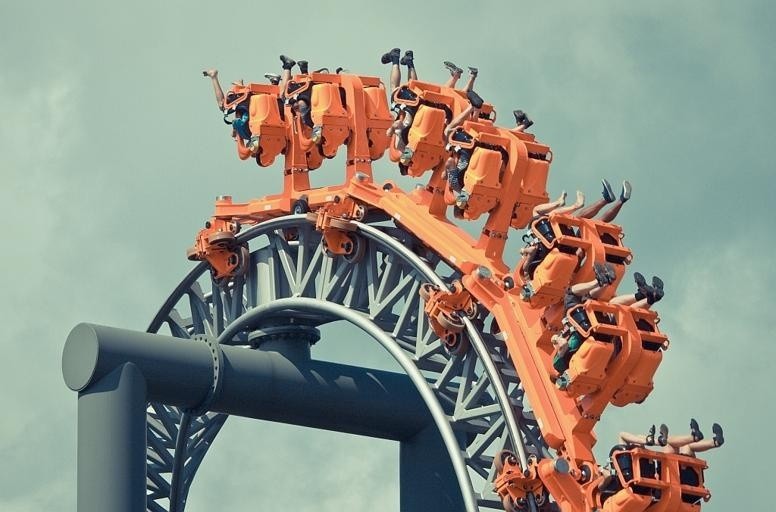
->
[199,69,219,78]
[523,112,534,130]
[400,50,414,67]
[312,68,329,74]
[230,79,244,87]
[601,177,617,203]
[620,179,632,203]
[279,55,296,69]
[262,72,282,84]
[513,109,526,122]
[467,65,480,77]
[651,275,665,301]
[465,88,485,108]
[335,66,343,75]
[381,47,402,65]
[297,59,308,75]
[633,271,654,298]
[443,60,464,80]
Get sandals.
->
[646,423,656,447]
[592,261,617,287]
[658,422,669,447]
[690,417,703,443]
[712,422,725,448]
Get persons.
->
[200,48,725,509]
